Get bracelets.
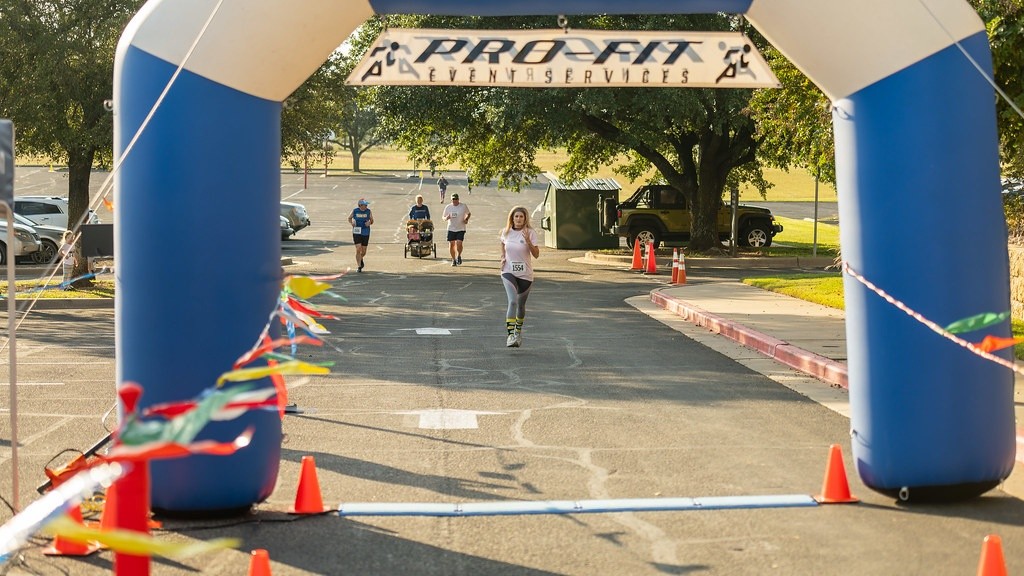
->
[466,218,468,220]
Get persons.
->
[89,208,98,280]
[348,199,374,272]
[500,206,540,347]
[409,195,430,221]
[442,194,471,266]
[58,231,76,291]
[431,168,435,177]
[466,168,473,195]
[438,175,449,204]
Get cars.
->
[0,220,43,266]
[11,212,70,264]
[278,201,311,239]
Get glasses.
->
[452,198,457,200]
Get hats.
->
[451,194,459,200]
[358,199,370,205]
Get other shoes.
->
[361,260,364,268]
[451,259,456,266]
[357,267,361,273]
[457,256,462,265]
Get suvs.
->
[602,184,784,251]
[12,195,101,231]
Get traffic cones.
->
[44,481,102,558]
[814,443,860,503]
[640,240,649,273]
[671,249,693,286]
[247,548,273,576]
[628,238,643,271]
[645,242,661,274]
[667,247,679,284]
[976,535,1009,576]
[287,455,338,516]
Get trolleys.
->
[403,218,437,262]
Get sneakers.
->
[506,333,518,346]
[514,333,522,346]
